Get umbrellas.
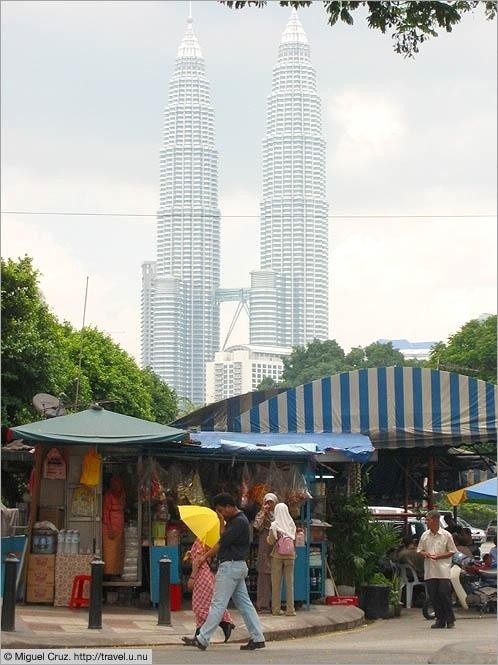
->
[175,504,221,548]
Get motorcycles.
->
[422,550,497,619]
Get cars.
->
[361,506,497,547]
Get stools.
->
[69,575,92,609]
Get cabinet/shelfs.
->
[309,541,326,598]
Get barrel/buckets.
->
[361,584,389,618]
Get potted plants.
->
[325,493,402,620]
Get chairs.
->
[398,564,429,610]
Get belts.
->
[221,558,244,563]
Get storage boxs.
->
[26,553,55,604]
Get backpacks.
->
[276,534,295,556]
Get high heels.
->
[223,622,235,643]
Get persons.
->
[405,512,497,569]
[266,503,298,617]
[181,531,235,646]
[253,491,279,614]
[385,513,497,617]
[181,494,265,652]
[415,509,456,629]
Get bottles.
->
[311,570,322,591]
[32,529,54,553]
[58,529,80,555]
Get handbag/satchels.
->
[206,554,220,575]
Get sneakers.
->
[240,639,267,651]
[258,608,271,614]
[447,623,456,628]
[182,636,207,651]
[431,621,446,628]
[286,611,296,616]
[272,609,285,616]
